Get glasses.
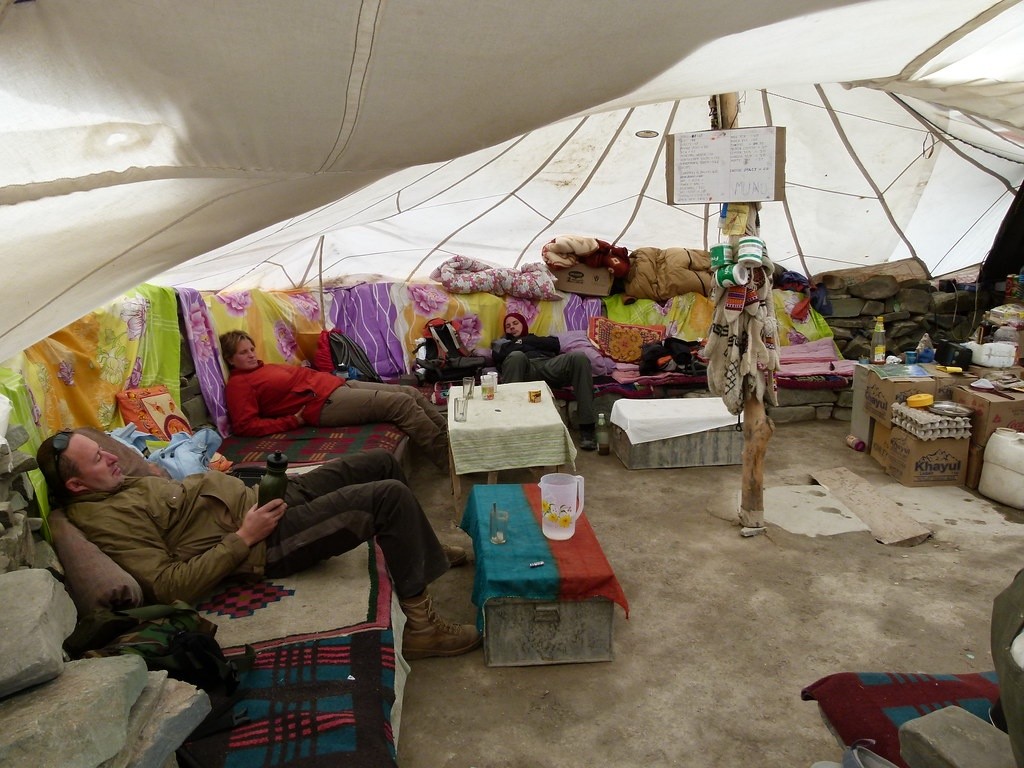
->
[52,431,73,483]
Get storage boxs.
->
[852,358,1024,489]
[550,260,614,297]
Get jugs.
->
[538,472,585,540]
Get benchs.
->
[1,265,852,768]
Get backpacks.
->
[425,318,485,370]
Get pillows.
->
[116,384,193,443]
[588,316,665,360]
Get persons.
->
[219,330,450,476]
[37,433,480,661]
[491,313,598,449]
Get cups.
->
[858,357,869,364]
[490,510,508,544]
[454,398,468,423]
[463,377,475,400]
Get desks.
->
[612,400,752,470]
[461,483,629,669]
[447,380,579,499]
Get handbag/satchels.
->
[173,632,239,697]
[329,332,381,381]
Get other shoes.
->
[843,739,900,768]
[579,424,597,449]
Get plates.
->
[927,401,975,416]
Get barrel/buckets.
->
[993,325,1021,366]
[978,427,1024,510]
[981,343,1015,368]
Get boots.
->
[402,588,482,660]
[441,543,465,566]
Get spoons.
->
[467,376,474,396]
[493,502,503,541]
[460,401,465,419]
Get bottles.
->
[869,317,886,365]
[906,351,917,365]
[335,363,349,381]
[487,372,498,393]
[258,449,289,509]
[480,375,494,400]
[917,346,935,363]
[845,434,865,452]
[596,413,610,456]
